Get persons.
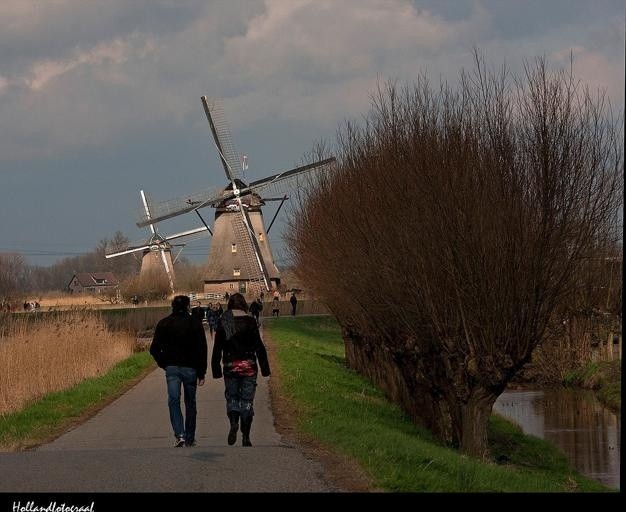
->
[271,296,281,316]
[149,295,208,447]
[191,301,224,341]
[290,293,297,316]
[23,300,41,313]
[131,294,138,308]
[211,293,270,446]
[248,298,263,329]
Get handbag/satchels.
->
[223,354,257,377]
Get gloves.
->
[264,376,270,381]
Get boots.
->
[240,414,253,447]
[227,410,240,445]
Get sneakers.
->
[173,435,199,448]
